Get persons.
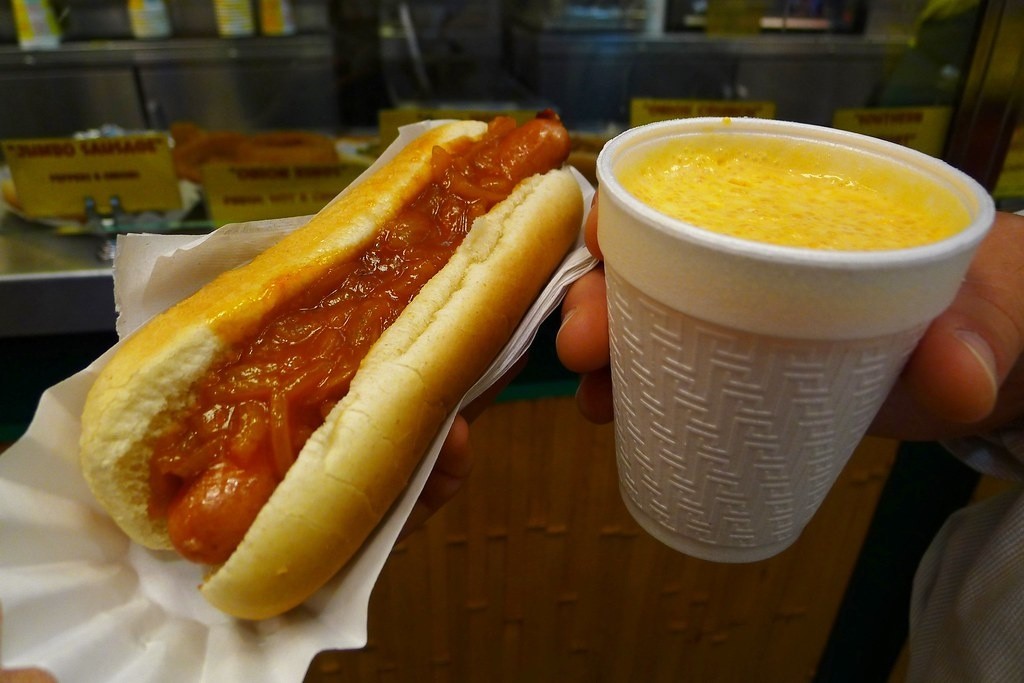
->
[0,184,1024,683]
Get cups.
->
[594,116,996,563]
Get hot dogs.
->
[77,109,583,620]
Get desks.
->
[0,394,907,683]
